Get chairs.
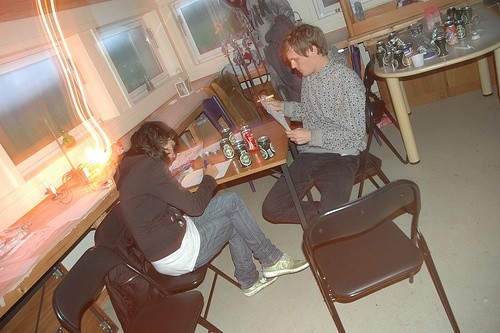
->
[93,202,242,333]
[302,179,462,333]
[52,245,224,333]
[203,44,397,199]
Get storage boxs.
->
[175,79,195,98]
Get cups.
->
[412,53,424,68]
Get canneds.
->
[433,6,472,58]
[240,125,258,153]
[257,136,276,161]
[376,32,414,70]
[220,138,235,159]
[232,139,253,168]
[222,128,235,144]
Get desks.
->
[373,9,500,166]
[171,117,309,229]
[0,51,218,333]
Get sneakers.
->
[242,271,279,296]
[263,254,311,278]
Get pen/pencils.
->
[204,161,207,169]
[256,94,275,103]
